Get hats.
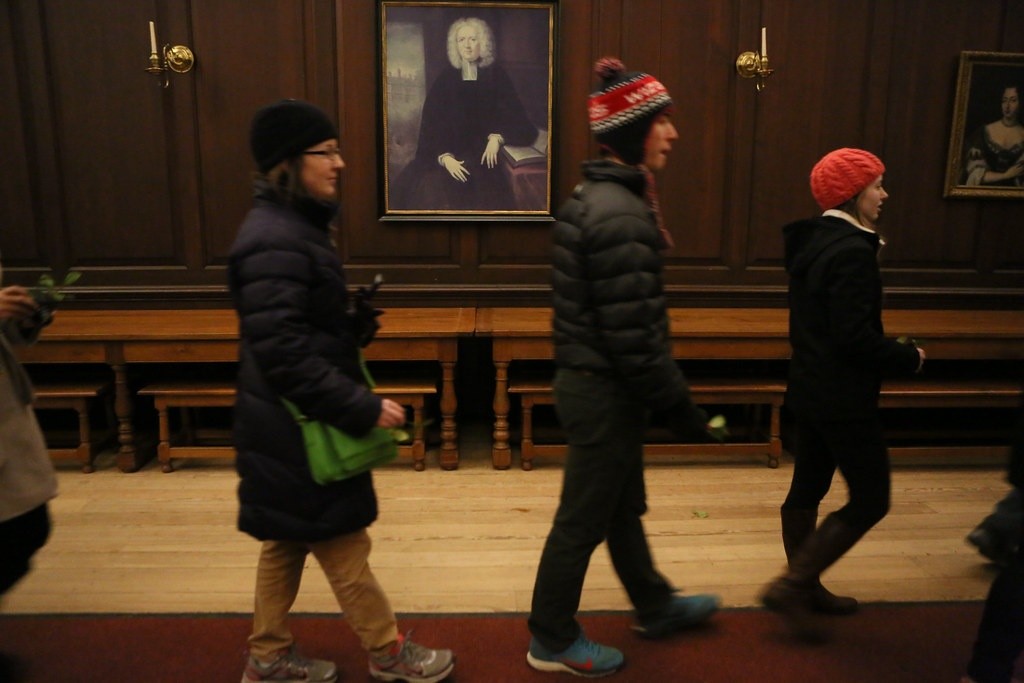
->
[250,98,338,175]
[811,147,886,210]
[587,57,672,165]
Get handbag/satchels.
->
[302,405,399,485]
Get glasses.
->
[300,147,341,161]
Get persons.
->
[961,433,1024,683]
[526,57,721,676]
[755,148,926,646]
[0,286,61,595]
[226,102,456,683]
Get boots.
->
[781,504,859,615]
[759,513,861,647]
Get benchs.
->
[503,378,786,466]
[134,376,439,475]
[877,378,1023,409]
[31,378,117,472]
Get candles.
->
[761,27,766,58]
[149,20,156,52]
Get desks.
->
[474,309,1024,468]
[25,304,474,468]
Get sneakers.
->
[635,590,721,639]
[365,633,458,683]
[527,623,623,678]
[240,647,338,683]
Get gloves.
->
[351,304,385,351]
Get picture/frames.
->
[377,1,559,223]
[941,46,1024,197]
